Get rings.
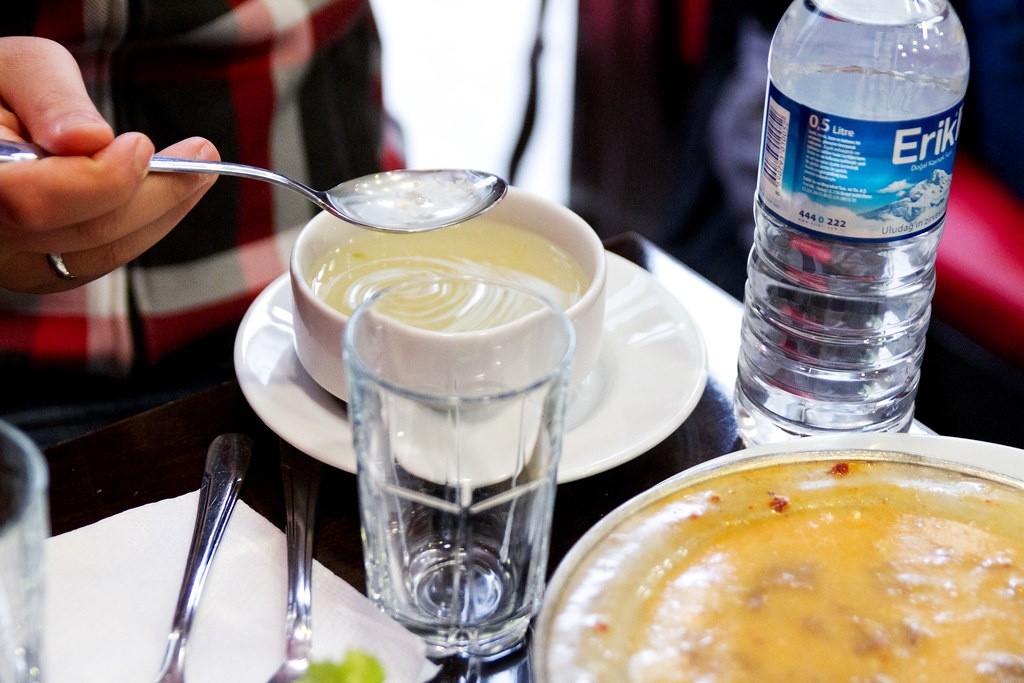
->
[47,252,80,283]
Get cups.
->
[337,270,578,674]
[2,428,51,683]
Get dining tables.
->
[0,228,951,682]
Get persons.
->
[1,0,408,441]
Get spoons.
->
[1,134,508,233]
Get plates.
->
[537,435,1021,683]
[43,485,446,683]
[231,250,715,515]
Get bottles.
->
[733,2,969,450]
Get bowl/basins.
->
[287,185,605,425]
